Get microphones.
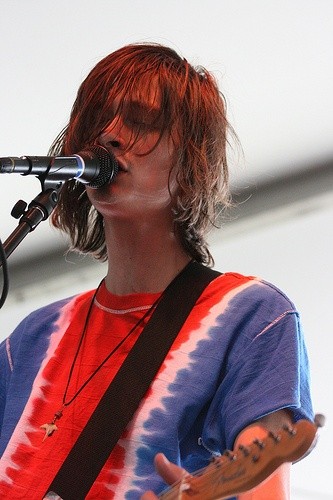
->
[0,143,119,191]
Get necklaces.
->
[39,257,196,443]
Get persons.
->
[0,42,319,500]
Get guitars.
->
[156,414,325,500]
